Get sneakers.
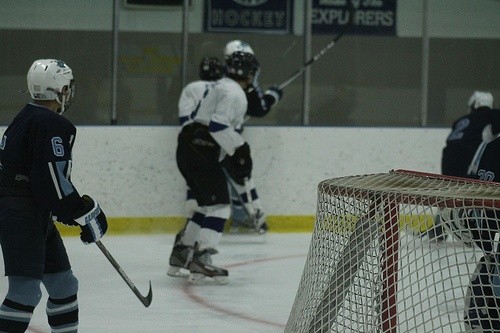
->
[188,244,229,285]
[165,218,198,278]
[229,217,268,234]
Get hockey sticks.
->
[223,169,265,233]
[404,221,434,238]
[244,0,355,125]
[97,239,153,309]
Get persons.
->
[0,59,107,333]
[442,88,500,333]
[165,39,284,284]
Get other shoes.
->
[418,227,448,241]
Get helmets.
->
[223,38,254,62]
[199,56,225,82]
[27,59,76,113]
[225,52,261,87]
[469,90,496,114]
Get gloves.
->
[263,87,283,108]
[228,141,252,186]
[74,195,108,245]
[55,199,78,227]
